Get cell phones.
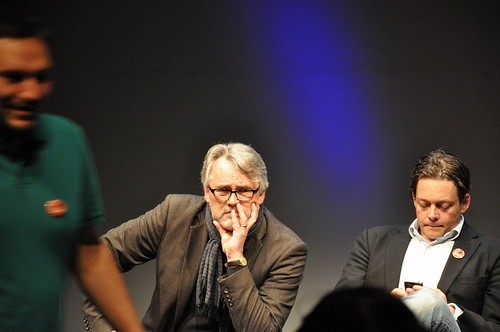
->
[405,282,423,291]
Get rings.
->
[240,223,247,227]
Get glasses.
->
[208,182,261,203]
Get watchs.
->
[225,257,247,267]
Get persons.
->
[0,19,147,332]
[80,143,307,332]
[334,149,500,332]
